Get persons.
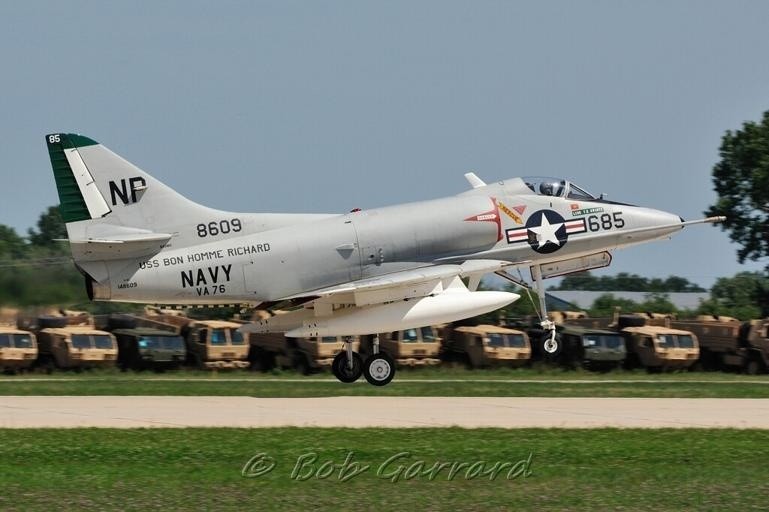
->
[539,180,554,196]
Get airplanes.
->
[45,133,727,386]
